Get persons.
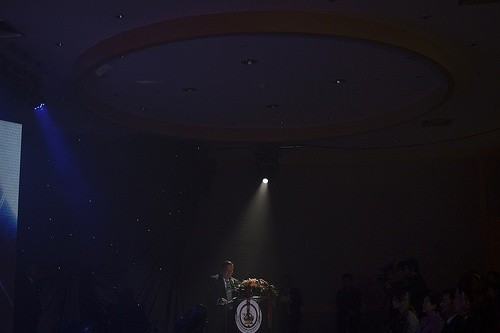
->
[335,273,363,333]
[210,261,239,333]
[275,274,305,332]
[382,258,500,333]
[177,303,207,333]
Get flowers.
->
[235,277,278,298]
